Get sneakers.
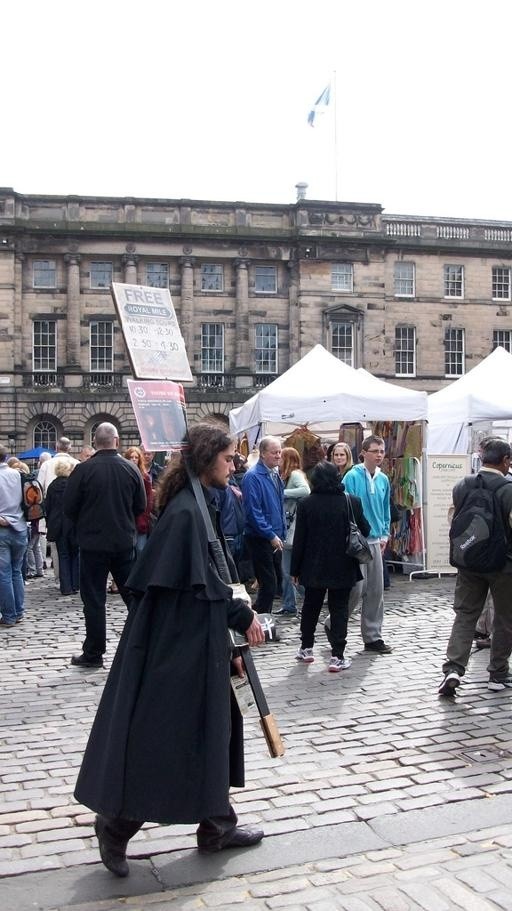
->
[0,614,23,627]
[267,607,511,693]
[198,828,265,856]
[93,817,129,875]
[24,561,79,595]
[71,651,104,667]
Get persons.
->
[290,460,371,672]
[324,435,393,653]
[201,411,242,558]
[61,421,148,665]
[330,442,355,478]
[433,433,512,696]
[242,436,287,614]
[0,436,95,627]
[270,446,313,616]
[106,446,164,594]
[72,419,266,880]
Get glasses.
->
[366,449,385,454]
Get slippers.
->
[107,587,120,593]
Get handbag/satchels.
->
[344,521,373,563]
[281,499,299,550]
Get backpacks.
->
[20,472,48,522]
[449,475,512,574]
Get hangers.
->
[281,425,322,441]
